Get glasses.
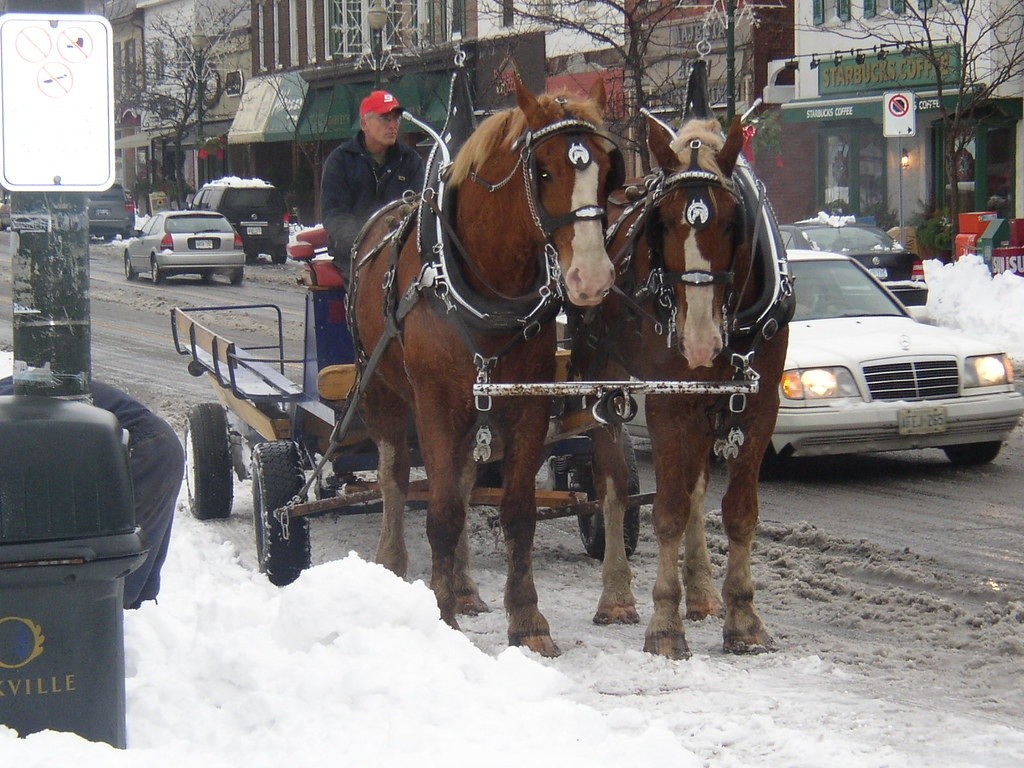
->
[370,113,402,125]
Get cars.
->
[87,184,135,242]
[0,199,11,231]
[624,223,1023,480]
[124,209,246,286]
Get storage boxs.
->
[955,233,981,262]
[958,212,997,234]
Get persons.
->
[322,90,424,288]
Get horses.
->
[345,77,794,661]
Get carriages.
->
[169,59,870,659]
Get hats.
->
[360,90,408,118]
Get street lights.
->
[192,23,208,184]
[367,0,388,90]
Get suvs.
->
[180,180,290,264]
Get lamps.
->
[834,55,842,67]
[810,58,820,70]
[855,54,865,65]
[785,61,798,70]
[877,50,889,61]
[902,149,909,167]
[901,47,912,58]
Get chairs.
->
[286,228,345,287]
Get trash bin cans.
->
[0,391,151,752]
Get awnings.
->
[227,72,452,143]
[781,84,984,123]
[116,119,233,151]
